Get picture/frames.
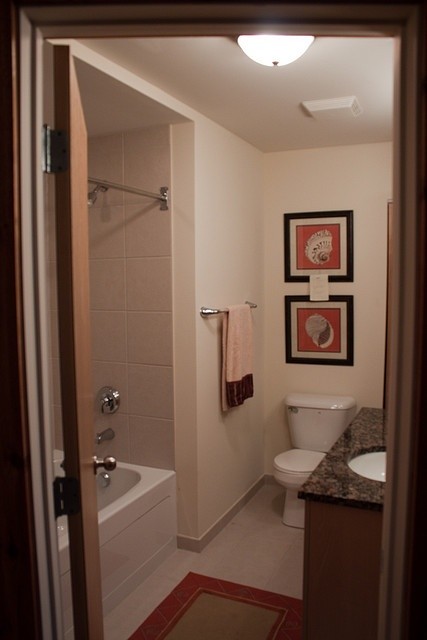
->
[283,293,352,366]
[283,209,355,281]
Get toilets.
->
[275,394,355,529]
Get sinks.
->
[345,441,387,489]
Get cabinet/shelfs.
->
[302,500,382,639]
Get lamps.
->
[234,35,315,69]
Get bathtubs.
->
[52,447,176,640]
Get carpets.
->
[130,569,303,635]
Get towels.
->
[218,304,253,415]
